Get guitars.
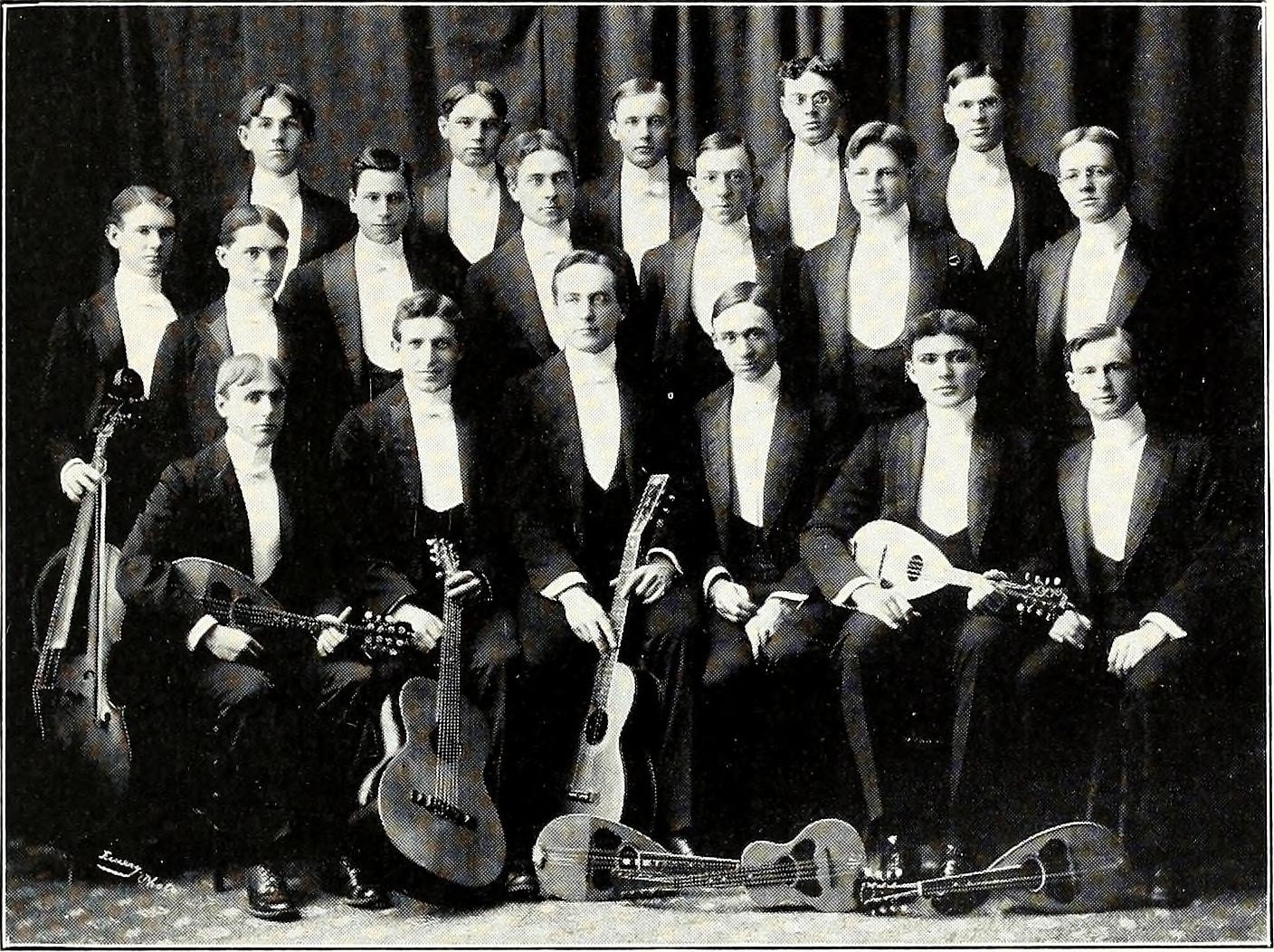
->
[153,552,417,659]
[513,469,685,841]
[662,815,872,916]
[852,816,1140,918]
[847,518,1077,623]
[528,811,741,905]
[346,530,510,913]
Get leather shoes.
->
[244,862,297,918]
[318,849,381,908]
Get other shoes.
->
[669,833,697,866]
[1147,854,1196,907]
[931,835,988,915]
[876,849,923,884]
[503,866,538,896]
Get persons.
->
[676,282,830,831]
[1005,326,1231,905]
[114,353,390,921]
[496,250,705,857]
[34,55,1206,549]
[800,308,1031,914]
[328,292,537,895]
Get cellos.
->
[25,362,149,835]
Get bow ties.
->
[624,179,664,202]
[239,466,271,484]
[414,396,454,422]
[459,176,491,197]
[361,257,403,278]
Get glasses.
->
[1057,167,1120,184]
